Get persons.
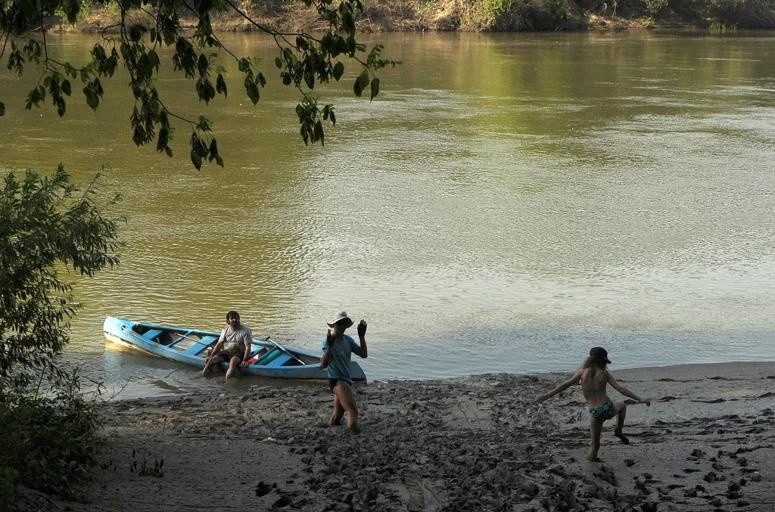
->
[315,309,368,433]
[198,309,253,380]
[531,343,651,462]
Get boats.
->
[103,316,368,382]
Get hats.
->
[590,347,612,364]
[327,310,354,329]
